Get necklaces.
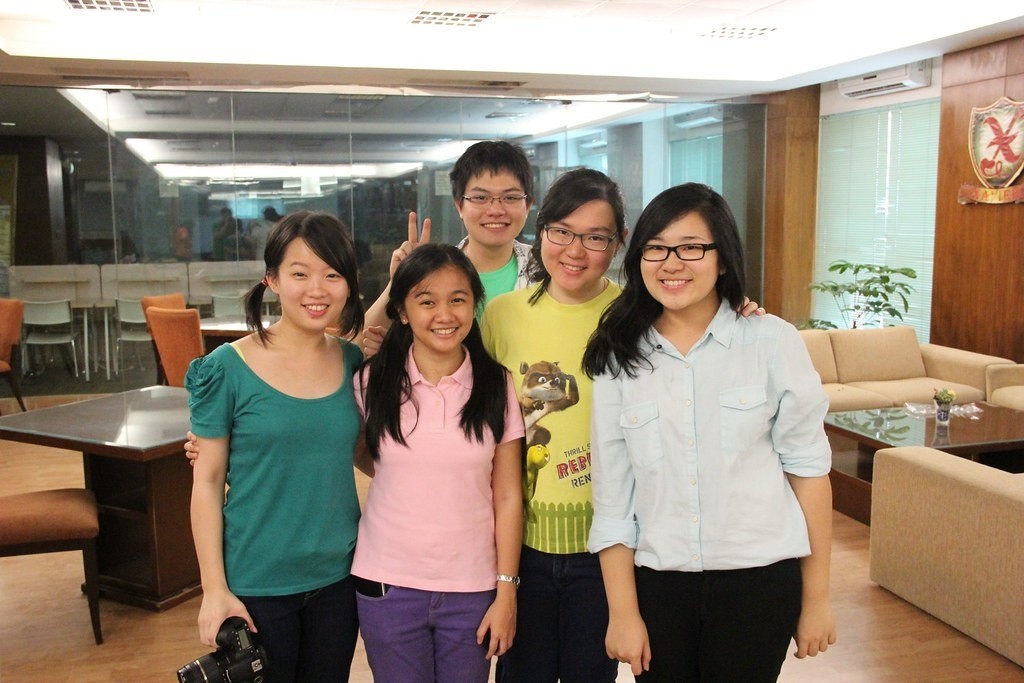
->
[601,278,607,292]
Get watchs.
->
[495,570,521,589]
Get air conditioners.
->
[580,133,607,149]
[837,58,932,98]
[673,106,724,129]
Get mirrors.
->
[0,83,766,311]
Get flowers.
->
[932,388,956,405]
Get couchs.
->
[801,325,1024,668]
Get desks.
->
[1,385,204,612]
[8,263,281,370]
[196,314,281,348]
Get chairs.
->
[20,297,79,376]
[0,298,28,415]
[145,307,207,389]
[212,294,249,317]
[113,297,157,373]
[1,489,105,645]
[140,291,188,387]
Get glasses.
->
[543,224,617,252]
[641,242,720,262]
[462,192,527,207]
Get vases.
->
[935,401,950,424]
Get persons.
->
[183,211,375,682]
[212,207,283,260]
[346,139,544,361]
[477,168,765,683]
[183,243,527,683]
[580,184,835,683]
[173,217,193,260]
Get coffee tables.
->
[824,401,1024,526]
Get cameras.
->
[177,615,270,683]
